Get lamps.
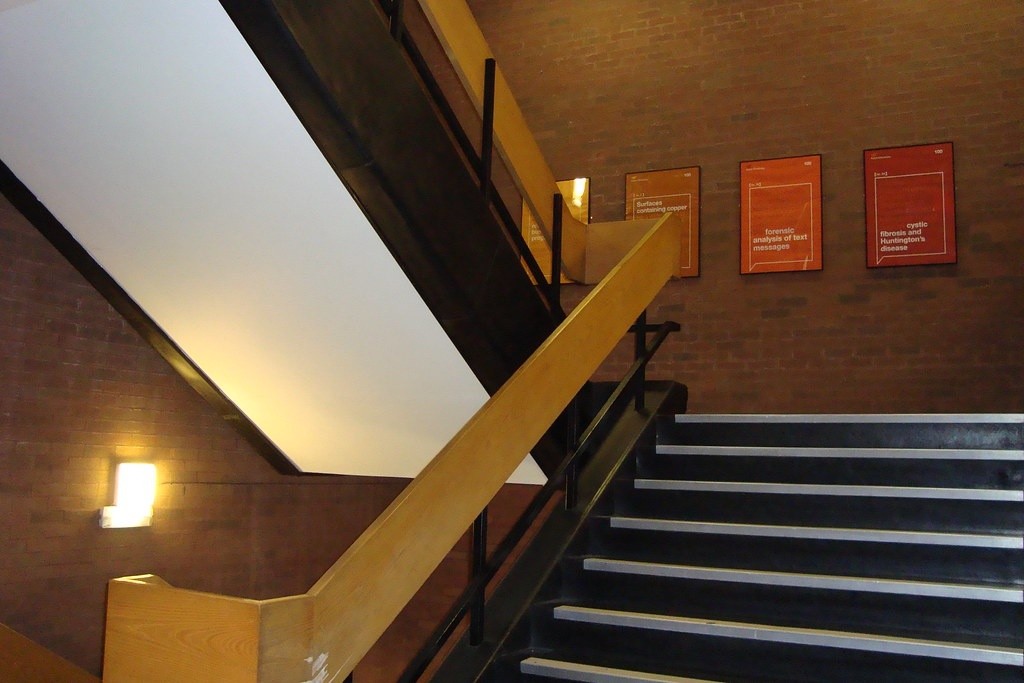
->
[99,460,156,527]
